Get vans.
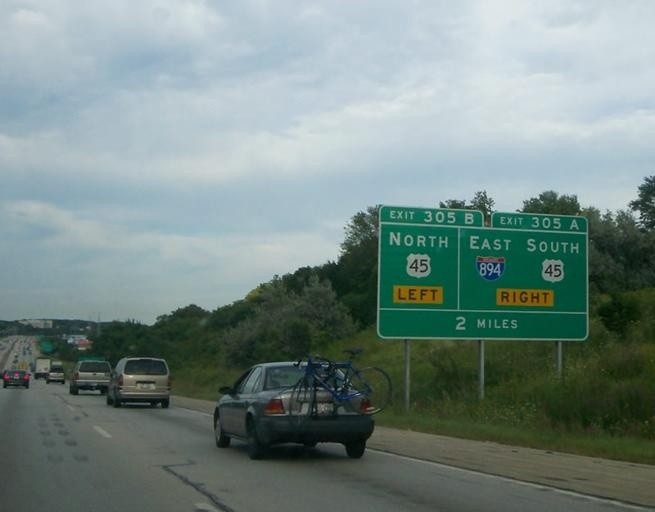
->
[2,356,173,408]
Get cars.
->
[213,362,375,459]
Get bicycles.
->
[288,347,393,428]
